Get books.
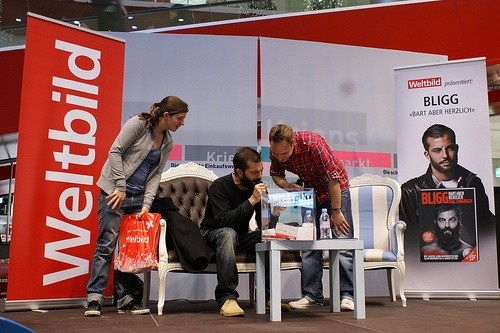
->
[419,187,479,261]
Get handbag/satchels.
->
[116,212,161,274]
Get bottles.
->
[319,208,332,239]
[304,210,315,241]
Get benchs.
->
[142,162,300,316]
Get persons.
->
[84,96,188,315]
[398,124,490,262]
[269,125,354,311]
[200,147,292,317]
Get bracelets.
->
[332,208,341,211]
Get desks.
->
[255,238,366,321]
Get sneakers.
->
[341,299,355,311]
[218,298,247,316]
[117,300,153,315]
[84,300,101,316]
[265,300,291,311]
[288,297,321,310]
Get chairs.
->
[320,173,408,308]
